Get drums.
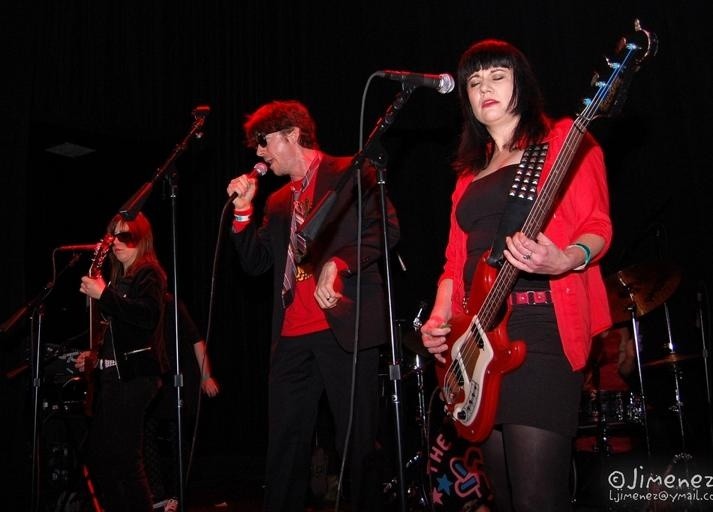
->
[582,388,645,427]
[419,414,580,511]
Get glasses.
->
[112,232,130,242]
[255,130,269,148]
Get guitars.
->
[83,232,111,415]
[436,19,659,444]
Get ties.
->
[281,154,321,309]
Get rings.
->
[524,253,532,259]
[326,296,331,301]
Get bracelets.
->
[567,243,591,271]
[233,214,252,222]
[201,373,212,379]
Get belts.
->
[99,346,154,371]
[505,290,553,305]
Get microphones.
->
[377,69,455,95]
[61,242,102,251]
[231,162,268,199]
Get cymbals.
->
[602,269,679,325]
[640,354,697,368]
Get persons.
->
[75,211,168,512]
[420,39,614,512]
[575,324,636,454]
[149,290,220,512]
[227,100,402,512]
